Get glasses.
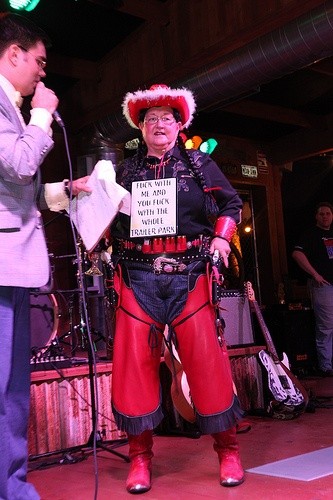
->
[142,114,177,125]
[12,43,47,68]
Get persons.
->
[88,85,246,494]
[0,10,93,500]
[291,203,333,377]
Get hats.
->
[122,84,196,130]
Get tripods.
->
[36,171,118,366]
[30,236,134,465]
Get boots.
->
[127,430,153,492]
[210,424,244,485]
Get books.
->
[65,158,132,253]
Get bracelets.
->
[64,180,75,201]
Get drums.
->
[29,288,73,361]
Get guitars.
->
[159,328,240,439]
[242,280,311,421]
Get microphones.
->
[53,108,66,131]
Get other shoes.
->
[321,370,333,376]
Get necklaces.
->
[145,157,171,170]
